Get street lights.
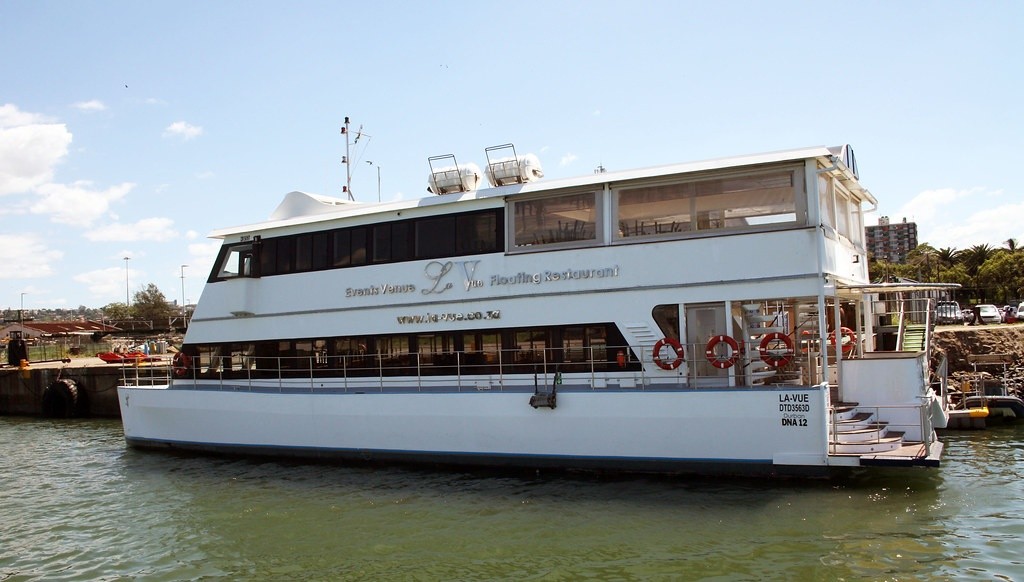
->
[123,257,131,306]
[181,264,189,328]
[21,292,28,331]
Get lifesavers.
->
[799,331,811,352]
[42,378,85,418]
[173,352,188,375]
[760,332,794,366]
[653,337,685,370]
[705,334,739,369]
[831,327,855,353]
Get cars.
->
[934,300,1024,324]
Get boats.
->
[117,116,950,481]
